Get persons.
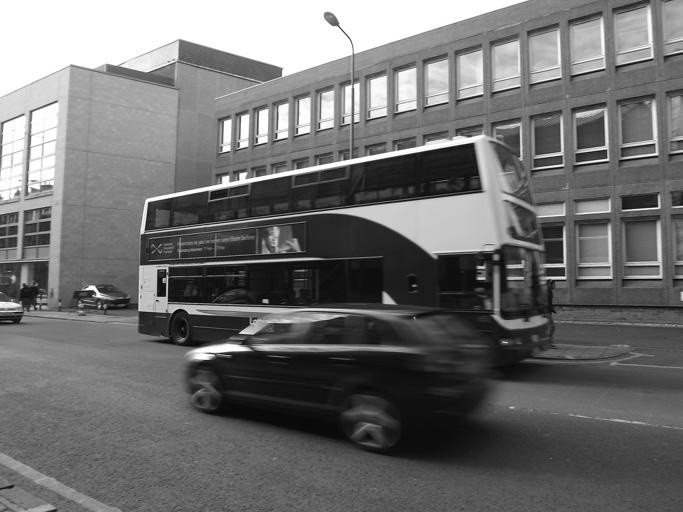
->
[262,227,301,253]
[19,283,40,312]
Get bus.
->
[136,133,555,366]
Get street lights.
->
[324,12,354,159]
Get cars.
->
[0,291,24,324]
[77,282,131,310]
[182,302,494,454]
